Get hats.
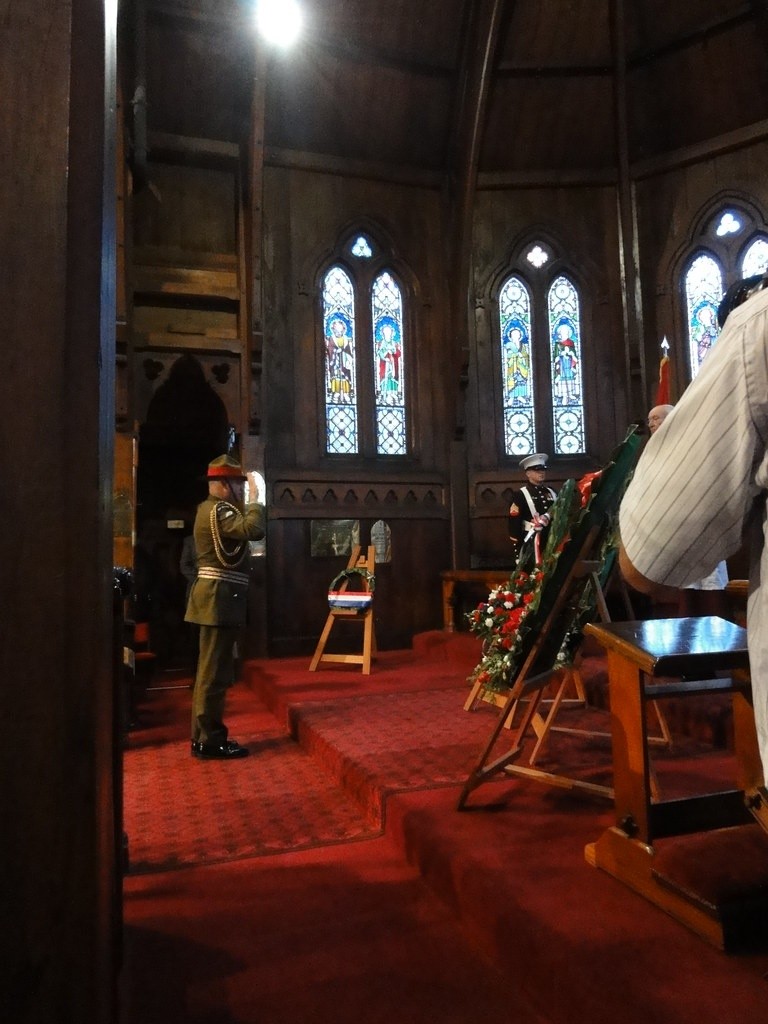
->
[519,452,549,473]
[207,454,248,481]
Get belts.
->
[197,567,250,587]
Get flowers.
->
[462,571,543,681]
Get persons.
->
[184,455,268,759]
[618,287,768,792]
[509,453,558,564]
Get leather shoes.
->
[190,740,250,760]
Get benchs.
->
[441,571,515,634]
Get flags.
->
[656,356,671,406]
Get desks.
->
[581,615,768,956]
